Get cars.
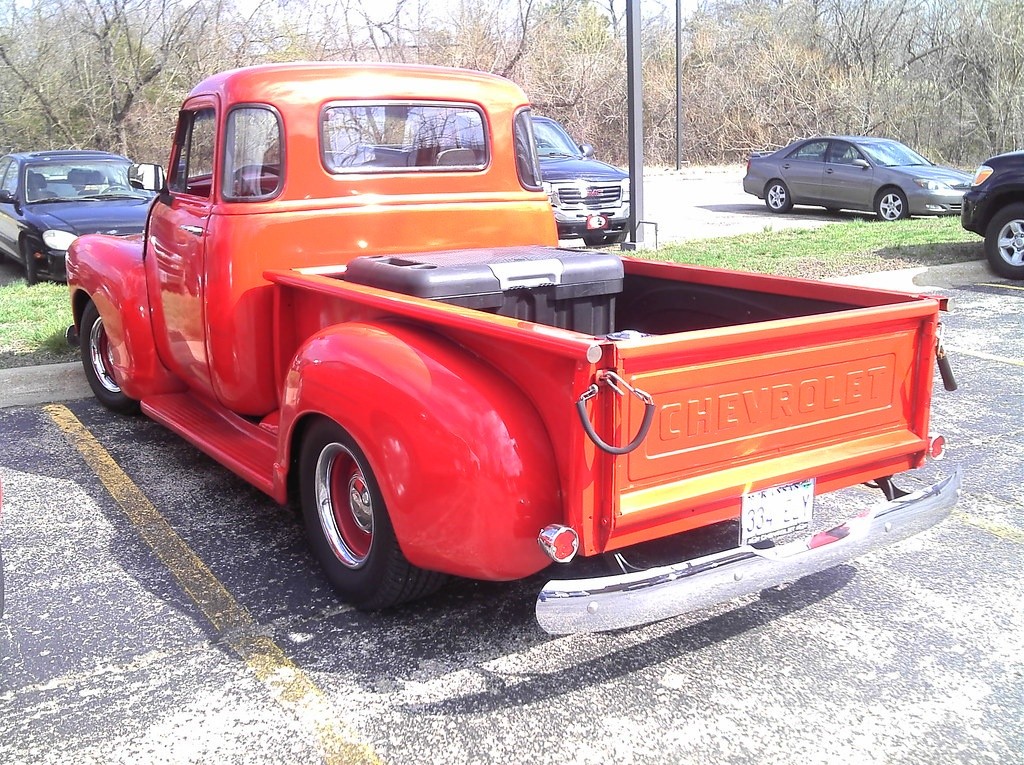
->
[962,150,1023,283]
[742,136,972,223]
[0,150,161,290]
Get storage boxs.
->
[345,247,624,335]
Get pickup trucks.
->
[357,112,631,248]
[63,63,960,636]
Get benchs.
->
[42,181,81,198]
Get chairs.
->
[435,149,475,164]
[27,170,57,200]
[85,171,112,195]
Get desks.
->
[798,144,868,166]
